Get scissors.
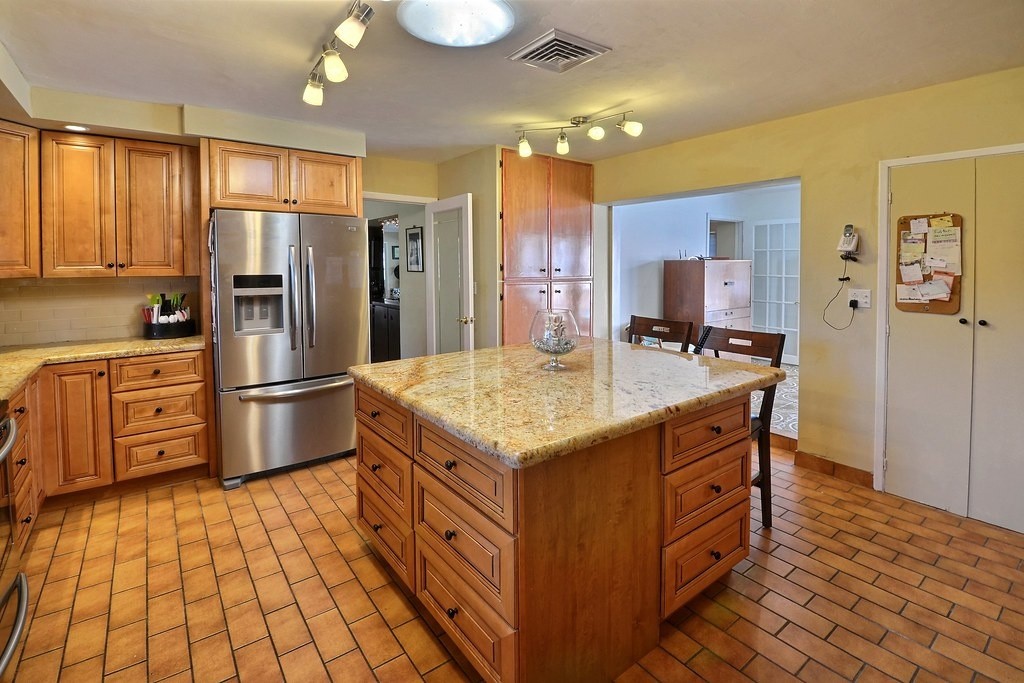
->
[170,292,184,309]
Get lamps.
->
[396,0,516,47]
[515,111,644,157]
[303,0,375,106]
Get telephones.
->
[836,224,859,252]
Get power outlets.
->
[848,289,871,307]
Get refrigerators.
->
[208,208,372,493]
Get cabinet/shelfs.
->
[664,259,751,364]
[369,301,400,360]
[210,137,358,217]
[0,120,209,551]
[501,148,594,345]
[353,380,751,682]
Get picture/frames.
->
[405,227,424,273]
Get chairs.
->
[699,325,786,528]
[627,316,693,352]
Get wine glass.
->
[529,308,580,371]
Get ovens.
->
[0,413,29,681]
